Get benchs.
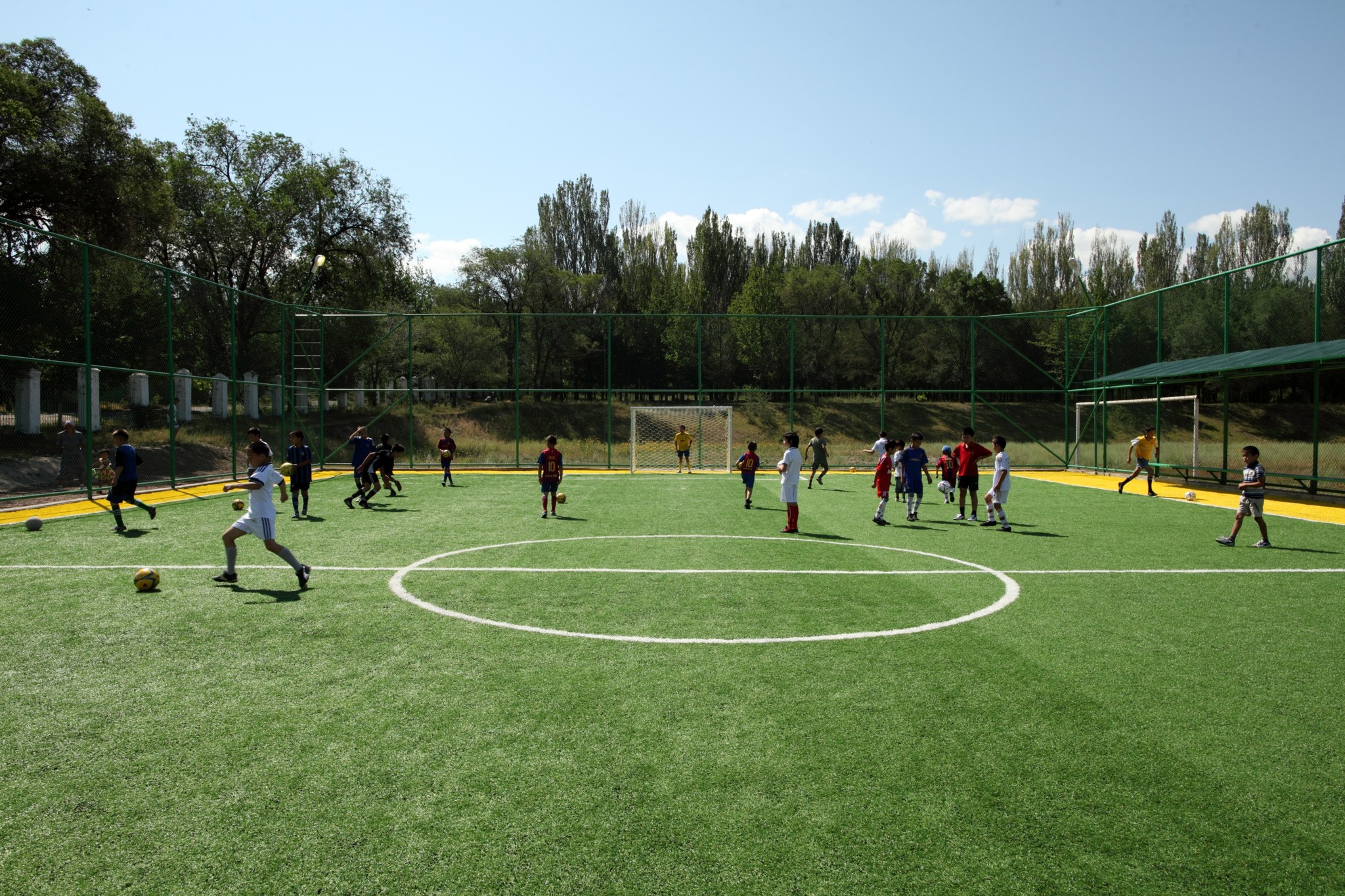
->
[1145,461,1345,495]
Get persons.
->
[861,427,1012,532]
[776,432,802,533]
[437,429,457,487]
[52,421,158,530]
[1118,425,1157,496]
[673,425,693,473]
[805,427,829,489]
[211,425,314,587]
[343,426,406,510]
[736,441,760,510]
[1215,446,1271,547]
[536,435,563,518]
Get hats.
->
[942,445,951,453]
[65,420,75,427]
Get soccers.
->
[937,480,951,494]
[280,462,294,477]
[232,499,245,511]
[849,467,856,473]
[738,461,743,471]
[25,515,43,531]
[443,450,451,458]
[556,493,566,504]
[383,481,392,489]
[1185,491,1196,501]
[133,568,160,592]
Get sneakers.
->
[550,510,555,516]
[447,482,453,486]
[541,510,547,518]
[356,497,361,502]
[912,512,919,520]
[953,514,965,520]
[943,501,949,503]
[292,512,299,518]
[949,491,955,502]
[967,514,977,521]
[344,498,354,509]
[300,509,307,515]
[688,470,691,473]
[995,527,1011,532]
[359,499,363,503]
[148,506,156,519]
[1148,491,1157,496]
[780,528,794,533]
[745,500,752,504]
[901,498,906,503]
[1118,482,1123,494]
[1252,540,1271,548]
[359,499,372,508]
[895,498,899,501]
[808,484,811,489]
[906,513,914,521]
[385,491,396,497]
[295,564,311,587]
[744,502,750,509]
[816,477,823,485]
[791,527,798,533]
[881,519,890,525]
[872,516,885,526]
[110,524,126,530]
[980,520,997,526]
[442,482,446,487]
[1216,534,1235,546]
[676,471,681,473]
[395,481,401,491]
[212,570,237,582]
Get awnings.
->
[1083,339,1345,384]
